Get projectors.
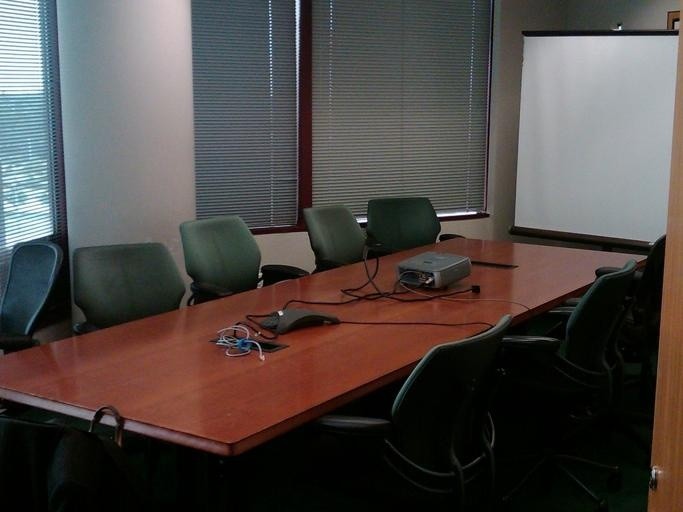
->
[397,250,472,291]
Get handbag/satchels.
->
[64,405,127,473]
